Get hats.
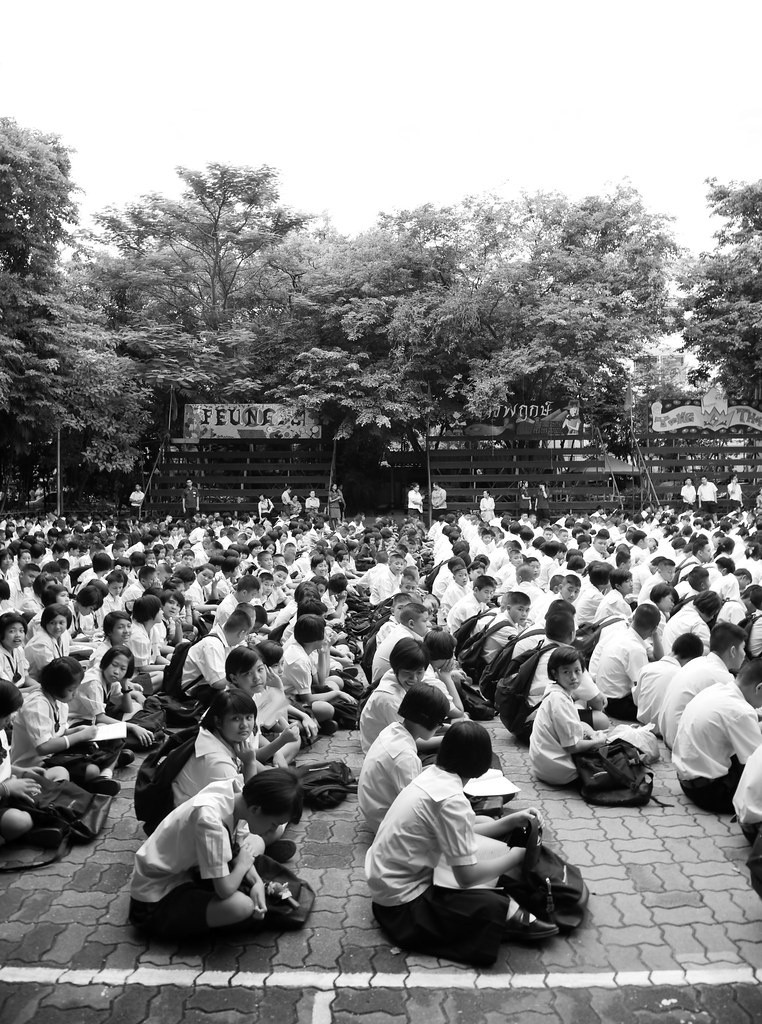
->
[462,769,520,797]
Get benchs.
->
[140,435,335,520]
[425,422,621,523]
[629,428,762,516]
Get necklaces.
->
[2,781,10,796]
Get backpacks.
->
[497,818,589,911]
[184,841,316,930]
[0,476,762,731]
[1,772,114,874]
[745,832,762,898]
[40,743,123,772]
[133,724,201,837]
[569,738,674,808]
[294,761,358,810]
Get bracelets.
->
[187,615,192,617]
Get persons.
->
[64,735,70,749]
[0,477,762,970]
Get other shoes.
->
[80,776,121,795]
[114,749,134,768]
[263,840,295,862]
[504,905,559,938]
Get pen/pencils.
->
[91,714,96,727]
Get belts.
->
[677,773,715,788]
[730,814,762,830]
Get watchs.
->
[185,608,192,612]
